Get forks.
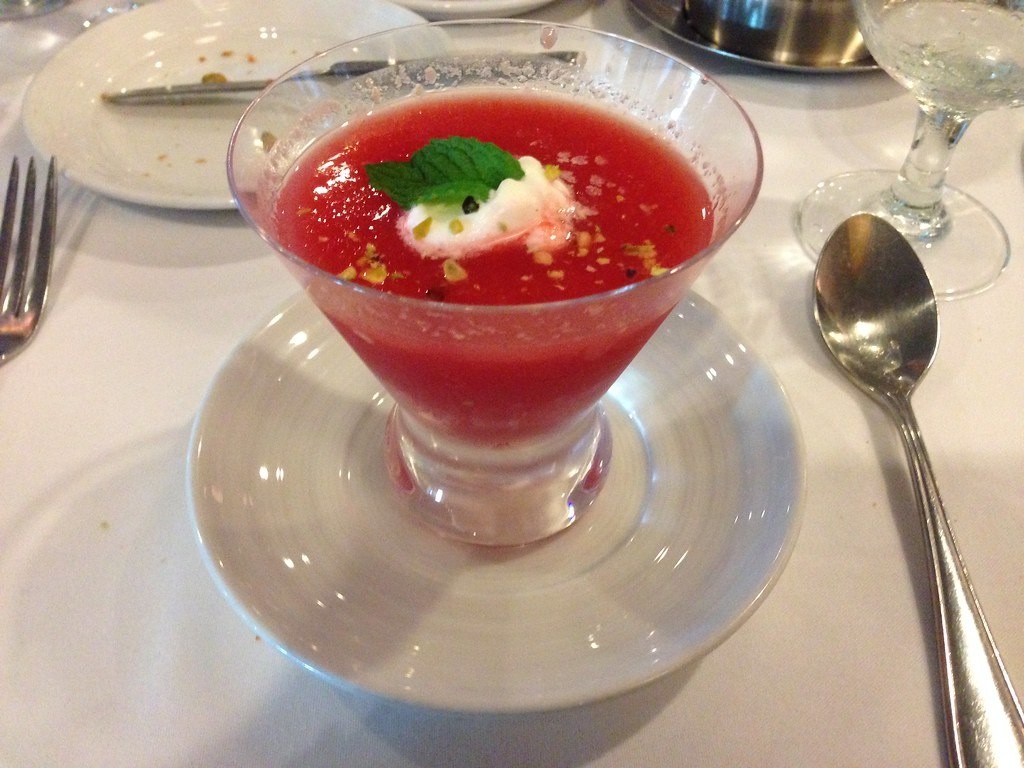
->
[0,153,59,364]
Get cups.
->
[228,17,763,542]
[685,0,873,63]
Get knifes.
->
[113,48,585,100]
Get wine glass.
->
[796,0,1024,298]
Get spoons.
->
[813,215,1024,768]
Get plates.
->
[188,285,807,714]
[393,0,550,21]
[627,0,880,71]
[20,0,459,211]
